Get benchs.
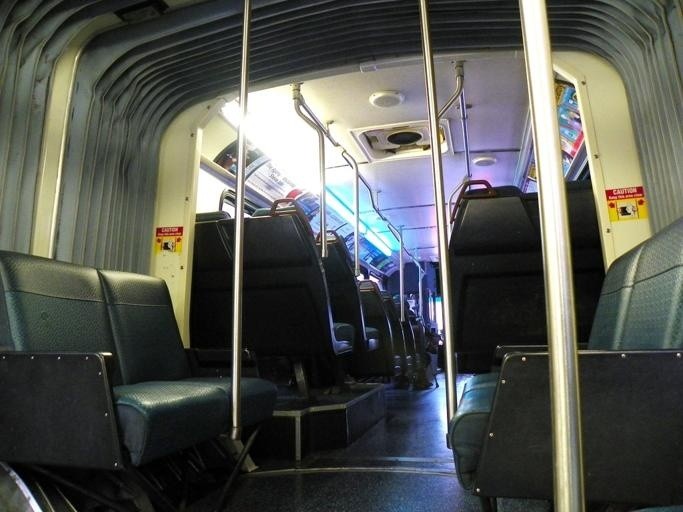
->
[446,216,683,512]
[0,250,277,512]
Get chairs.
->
[566,181,607,345]
[449,179,547,372]
